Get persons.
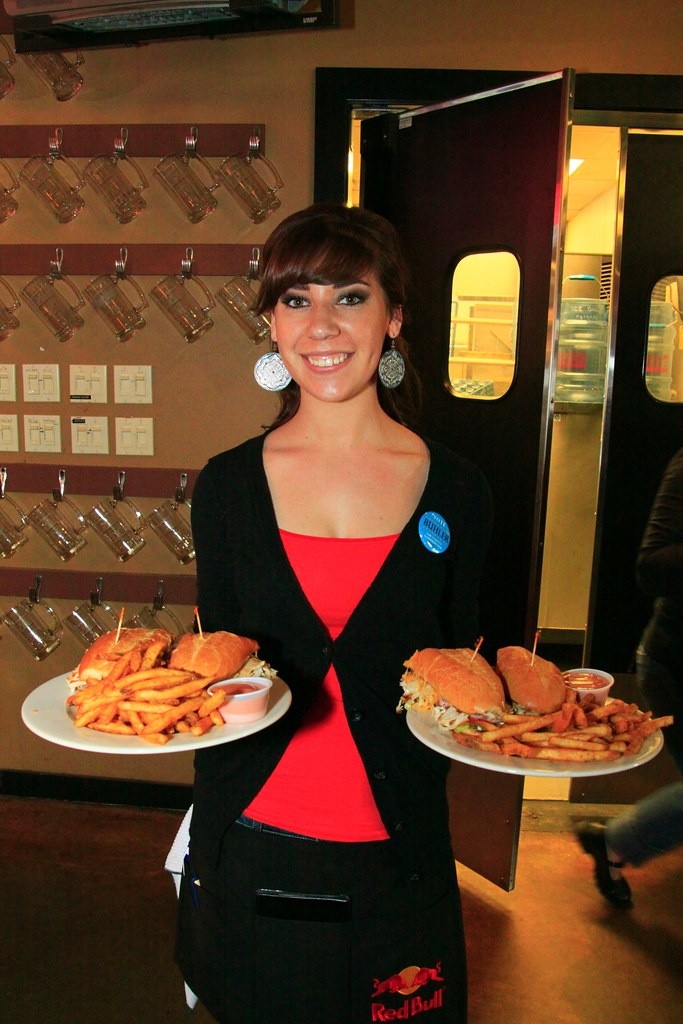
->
[577,446,683,910]
[174,204,510,1024]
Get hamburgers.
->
[395,648,505,732]
[493,646,566,715]
[67,626,176,690]
[166,630,278,687]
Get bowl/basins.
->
[562,668,615,706]
[206,677,273,725]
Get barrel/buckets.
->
[645,301,678,401]
[554,274,610,401]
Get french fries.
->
[67,641,228,746]
[449,687,673,761]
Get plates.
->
[405,696,664,777]
[21,667,292,754]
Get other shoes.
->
[573,821,632,906]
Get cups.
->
[1,599,63,662]
[15,47,86,101]
[215,273,271,345]
[82,152,149,224]
[215,151,285,224]
[82,272,150,342]
[19,272,86,343]
[83,494,146,563]
[144,498,196,566]
[0,158,20,223]
[124,605,183,660]
[152,151,222,224]
[62,599,119,650]
[0,35,17,100]
[0,276,21,342]
[27,495,89,562]
[19,152,85,224]
[0,494,30,559]
[149,273,216,344]
